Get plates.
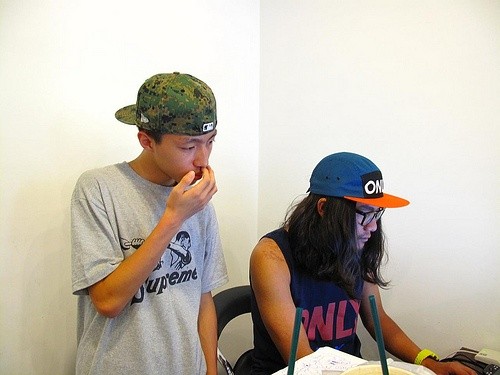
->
[339,364,414,375]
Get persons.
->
[247,152,480,375]
[70,71,229,375]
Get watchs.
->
[414,349,440,366]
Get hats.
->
[306,151,410,206]
[114,71,217,135]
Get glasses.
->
[346,205,385,226]
